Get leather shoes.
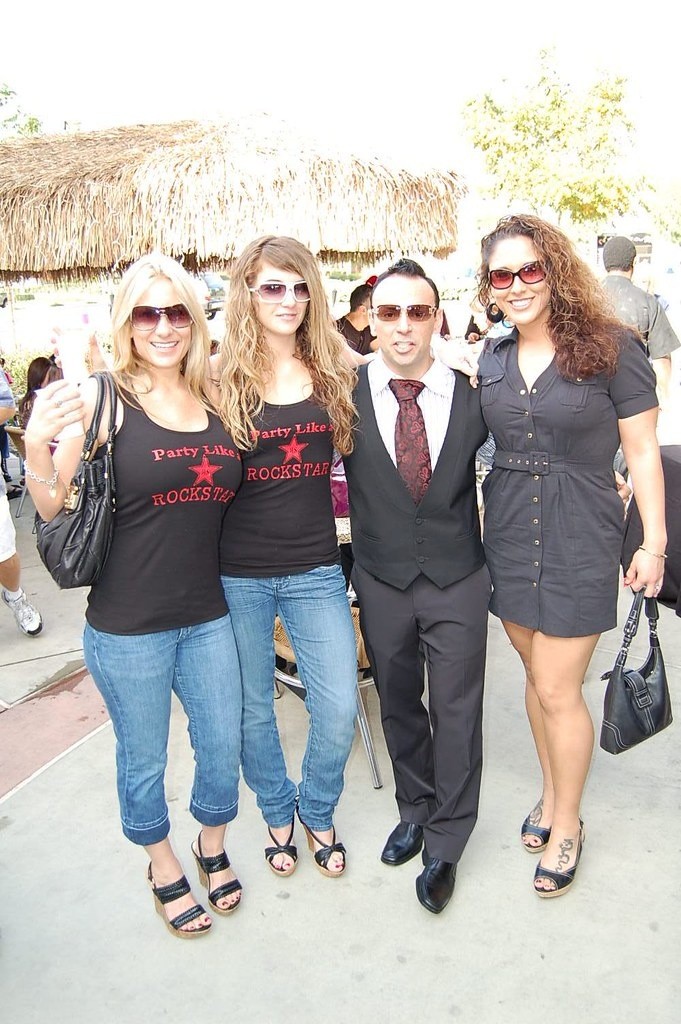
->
[416,846,457,914]
[380,820,426,864]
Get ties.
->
[388,380,433,507]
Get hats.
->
[603,236,637,270]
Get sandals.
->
[521,807,551,853]
[535,818,586,898]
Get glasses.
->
[486,263,547,290]
[370,302,438,323]
[128,302,194,332]
[250,281,311,304]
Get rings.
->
[653,584,659,590]
[54,397,64,406]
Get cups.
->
[35,385,84,442]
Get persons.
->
[0,236,681,635]
[339,259,633,915]
[24,254,243,937]
[458,213,670,899]
[203,235,478,878]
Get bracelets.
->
[638,544,667,561]
[23,459,60,500]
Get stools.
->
[272,607,388,789]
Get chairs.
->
[3,425,61,518]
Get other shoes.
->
[4,473,13,482]
[19,479,26,486]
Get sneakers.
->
[2,587,44,637]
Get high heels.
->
[295,794,348,878]
[264,814,298,878]
[145,859,212,940]
[190,830,244,917]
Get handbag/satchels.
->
[32,369,116,589]
[600,587,674,754]
[329,456,351,518]
